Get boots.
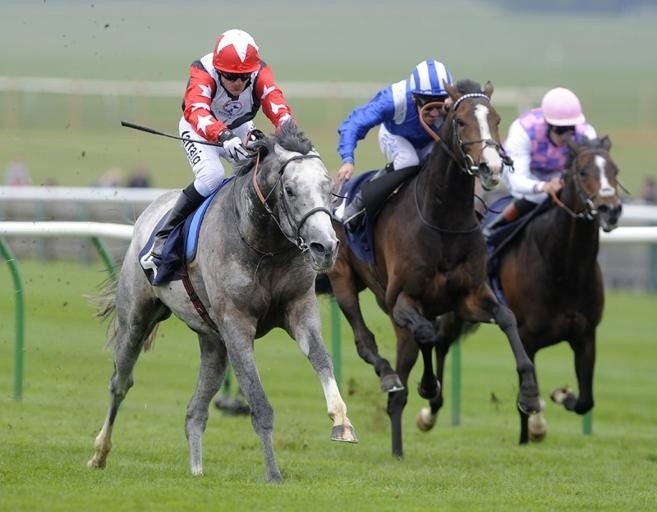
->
[342,163,391,230]
[150,189,195,260]
[483,196,537,242]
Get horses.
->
[415,134,622,443]
[314,77,542,458]
[82,117,358,488]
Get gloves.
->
[219,129,249,163]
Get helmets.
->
[541,87,586,126]
[410,59,452,96]
[212,28,261,74]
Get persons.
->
[332,58,453,231]
[149,28,298,269]
[479,86,597,248]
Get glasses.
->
[218,70,253,81]
[416,99,442,111]
[553,125,574,135]
